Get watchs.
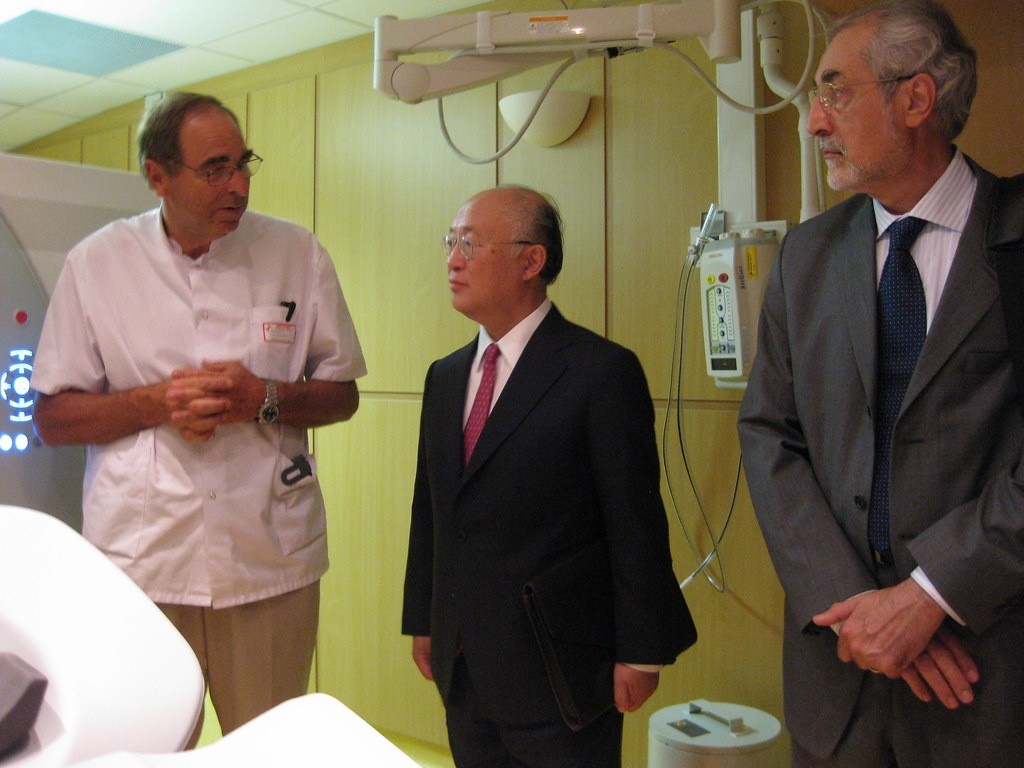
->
[254,378,280,426]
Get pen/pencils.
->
[279,300,296,323]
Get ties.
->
[463,343,501,469]
[868,217,927,552]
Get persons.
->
[401,186,698,768]
[736,0,1024,768]
[29,90,368,749]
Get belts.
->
[873,551,896,563]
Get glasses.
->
[161,149,263,188]
[442,234,536,257]
[808,74,918,107]
